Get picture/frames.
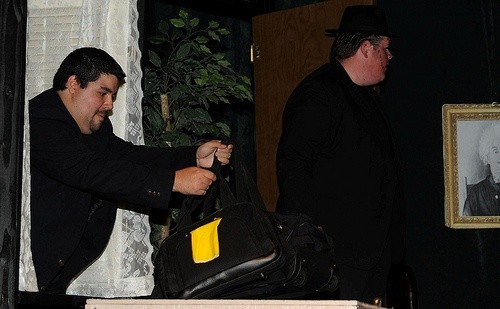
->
[442,103,500,229]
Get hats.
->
[323,4,395,35]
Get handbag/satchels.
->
[156,139,336,298]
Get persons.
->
[14,47,234,295]
[277,5,419,309]
[462,121,500,216]
